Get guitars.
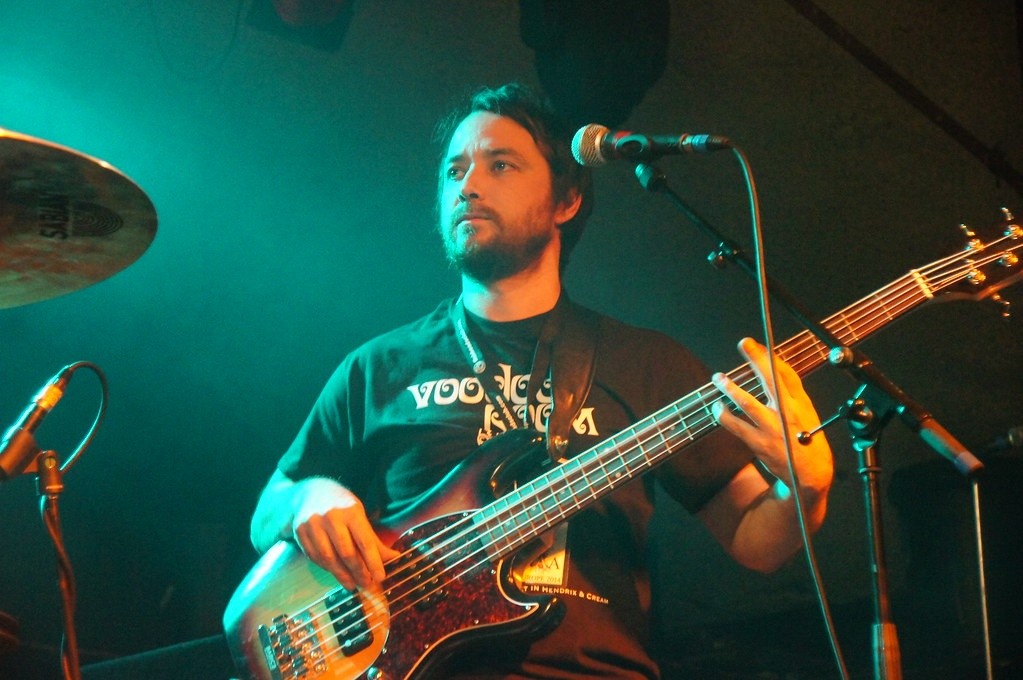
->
[220,206,1023,680]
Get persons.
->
[250,86,832,680]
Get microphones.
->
[0,365,74,482]
[571,122,734,167]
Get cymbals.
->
[0,127,159,310]
